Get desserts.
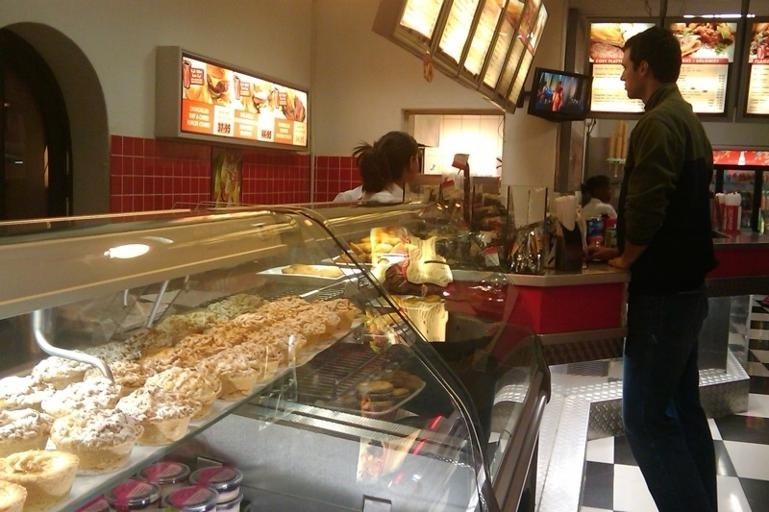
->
[0,225,410,512]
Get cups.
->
[715,203,744,236]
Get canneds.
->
[585,216,607,261]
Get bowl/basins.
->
[0,298,360,512]
[83,460,246,512]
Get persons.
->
[552,78,564,111]
[582,25,721,511]
[332,131,419,205]
[579,174,619,237]
[540,81,548,104]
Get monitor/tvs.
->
[527,66,593,121]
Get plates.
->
[321,255,405,267]
[256,264,366,281]
